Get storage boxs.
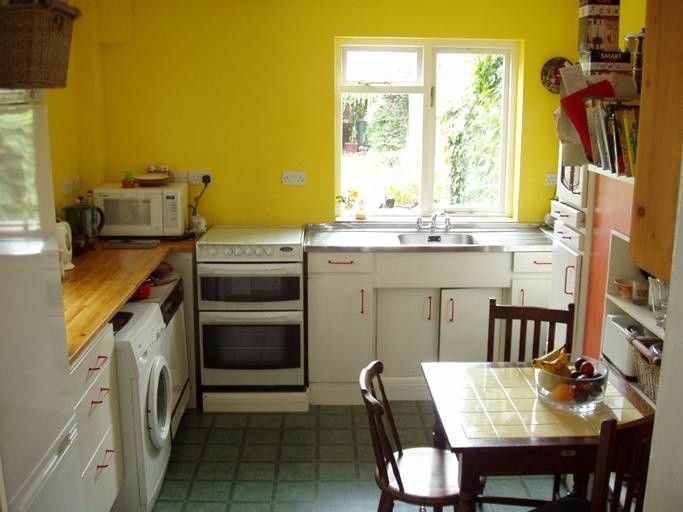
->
[579,4,620,16]
[579,50,634,78]
[578,15,620,53]
[579,0,621,7]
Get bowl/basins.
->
[613,278,632,299]
[533,355,612,410]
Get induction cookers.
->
[195,224,306,263]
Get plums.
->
[571,357,605,403]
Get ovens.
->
[195,261,310,415]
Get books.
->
[583,93,639,179]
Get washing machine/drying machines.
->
[108,301,174,512]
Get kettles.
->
[55,215,77,271]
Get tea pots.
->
[63,195,105,250]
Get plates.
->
[134,178,170,187]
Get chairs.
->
[357,356,487,512]
[529,414,658,512]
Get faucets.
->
[416,213,452,233]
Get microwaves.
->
[92,181,190,240]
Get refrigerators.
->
[0,89,89,512]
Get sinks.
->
[398,232,479,247]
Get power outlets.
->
[188,171,199,184]
[282,172,305,184]
[199,171,212,184]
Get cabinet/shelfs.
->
[69,322,124,512]
[304,164,590,412]
[600,229,673,410]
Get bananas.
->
[532,343,568,372]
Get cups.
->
[632,278,648,306]
[121,180,134,188]
[650,277,668,322]
[191,216,206,239]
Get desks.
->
[419,354,657,512]
